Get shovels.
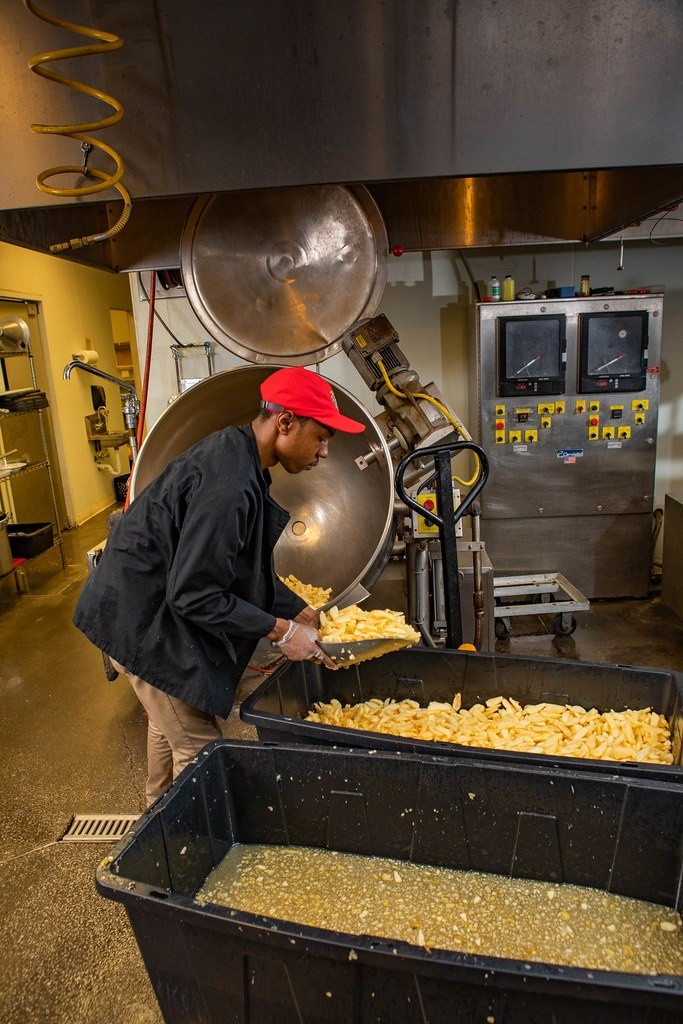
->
[270,636,416,670]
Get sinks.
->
[88,430,130,447]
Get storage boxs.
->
[236,642,683,785]
[92,732,683,1024]
[5,520,56,560]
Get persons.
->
[72,367,367,809]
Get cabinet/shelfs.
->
[0,339,69,595]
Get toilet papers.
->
[75,349,100,365]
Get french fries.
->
[274,573,675,767]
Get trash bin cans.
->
[114,472,130,503]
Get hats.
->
[260,368,366,434]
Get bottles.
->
[581,274,590,296]
[487,276,501,302]
[502,275,515,301]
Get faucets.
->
[97,406,107,423]
[62,361,140,397]
